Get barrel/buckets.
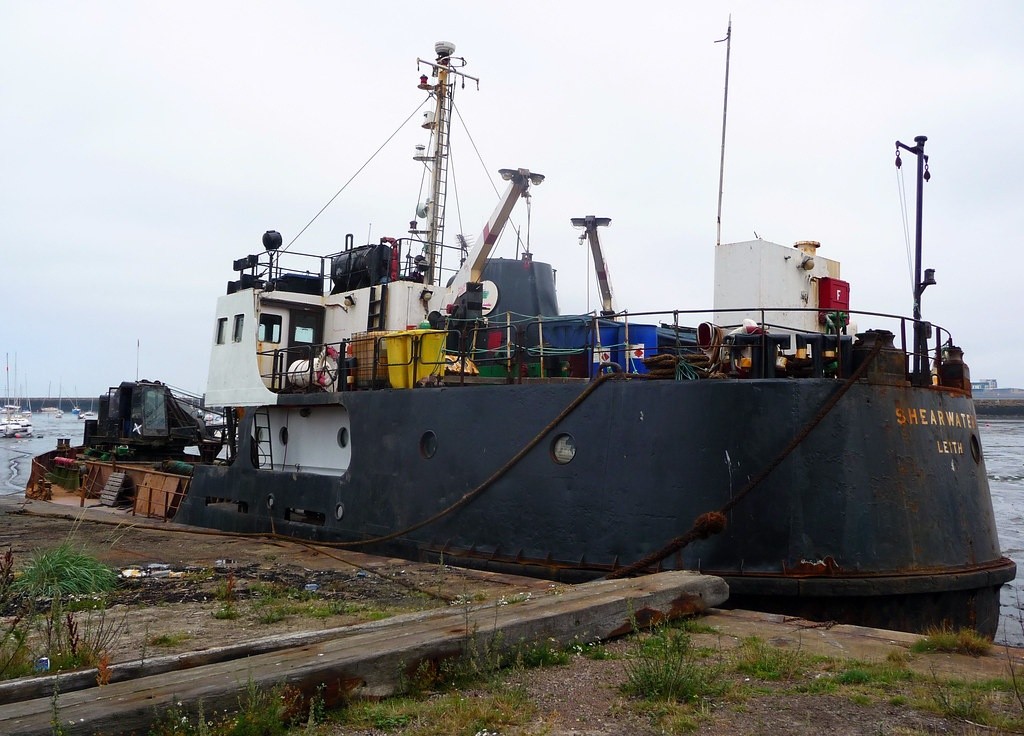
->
[618,324,658,374]
[587,325,616,377]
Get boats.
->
[21,39,1017,641]
[0,351,96,438]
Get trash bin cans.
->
[386,329,409,388]
[588,326,619,378]
[405,329,449,388]
[617,324,659,375]
